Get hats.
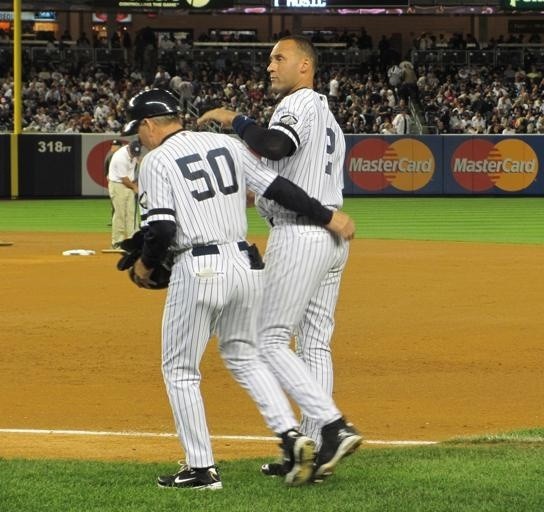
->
[130,140,141,156]
[110,139,123,146]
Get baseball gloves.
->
[118,230,174,287]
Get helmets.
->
[119,86,182,136]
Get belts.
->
[194,241,250,258]
[270,217,275,227]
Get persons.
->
[104,140,140,251]
[103,139,137,226]
[0,25,543,134]
[194,33,360,483]
[124,87,358,489]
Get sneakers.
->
[110,243,122,250]
[157,460,223,492]
[261,419,364,487]
